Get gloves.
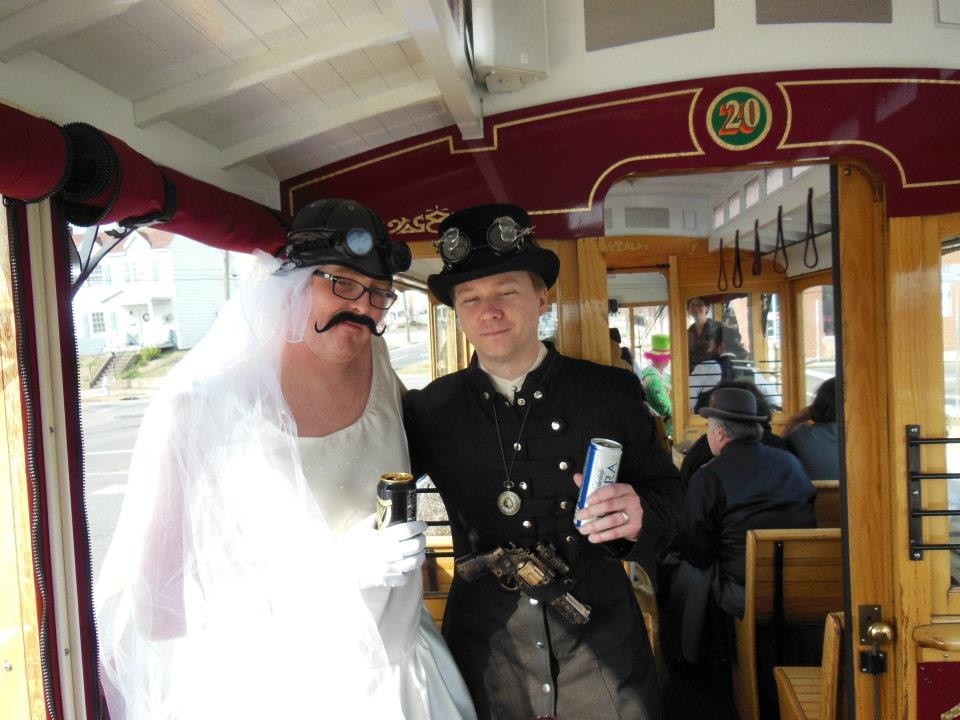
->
[333,511,428,590]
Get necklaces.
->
[493,402,532,516]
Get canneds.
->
[574,438,622,530]
[376,472,416,529]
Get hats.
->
[700,388,768,421]
[285,199,394,283]
[705,327,741,348]
[644,334,671,366]
[428,203,560,308]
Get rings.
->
[620,511,628,524]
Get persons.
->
[401,204,690,720]
[607,298,839,720]
[93,199,478,720]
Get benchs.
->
[730,478,846,719]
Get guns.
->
[455,539,592,626]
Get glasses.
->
[439,218,531,264]
[330,225,413,274]
[313,270,397,310]
